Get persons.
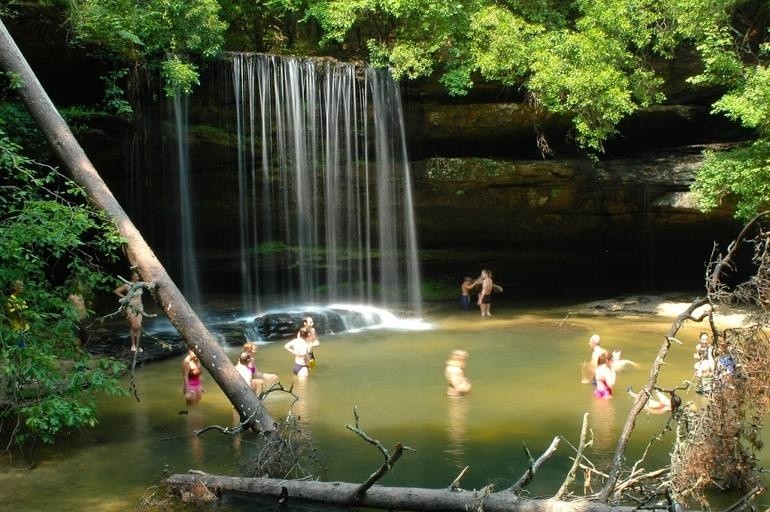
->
[234,352,253,389]
[444,349,471,397]
[284,327,310,382]
[297,316,320,368]
[66,281,88,345]
[461,268,503,317]
[580,331,736,416]
[113,269,144,353]
[7,280,32,350]
[236,342,278,389]
[182,346,208,406]
